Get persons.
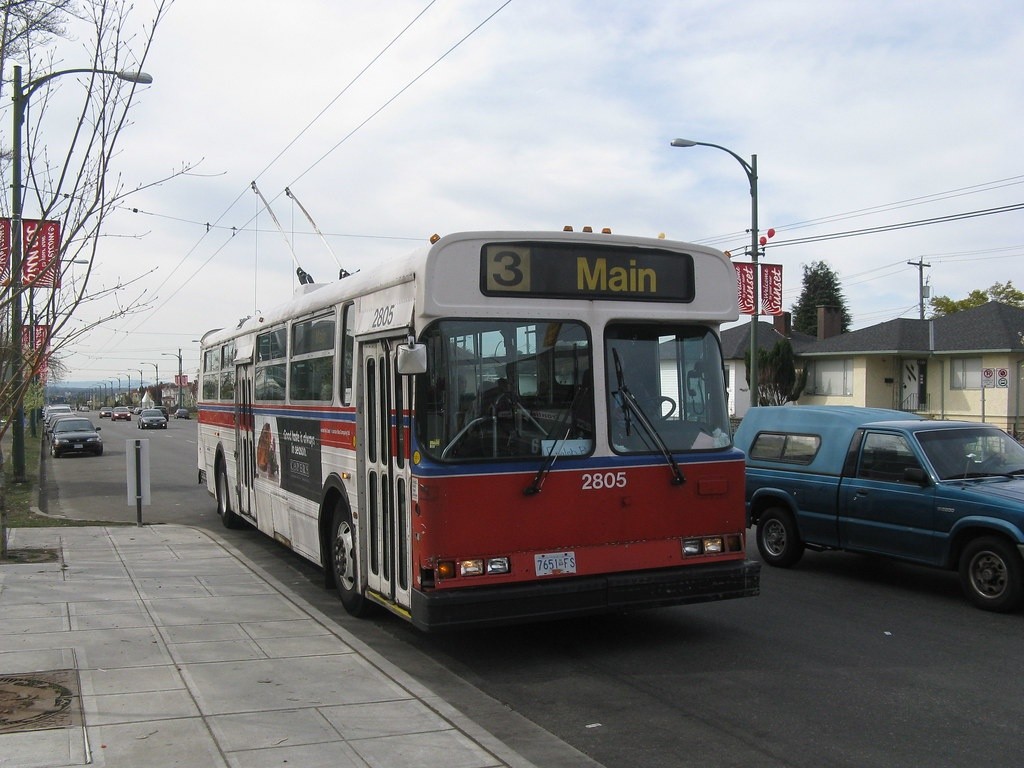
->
[933,438,1008,479]
[607,347,660,432]
[443,374,483,453]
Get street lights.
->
[139,361,159,405]
[108,376,121,399]
[669,137,761,408]
[161,348,183,408]
[116,372,131,406]
[126,368,143,405]
[86,379,114,406]
[11,62,155,486]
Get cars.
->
[99,406,113,419]
[79,406,83,411]
[174,408,190,420]
[82,407,89,412]
[151,406,169,422]
[42,403,103,457]
[137,409,168,429]
[133,407,142,414]
[126,406,134,413]
[111,407,131,421]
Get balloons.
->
[768,229,775,238]
[760,237,766,246]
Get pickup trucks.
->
[729,405,1024,612]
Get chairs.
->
[869,440,913,481]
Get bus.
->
[192,224,760,635]
